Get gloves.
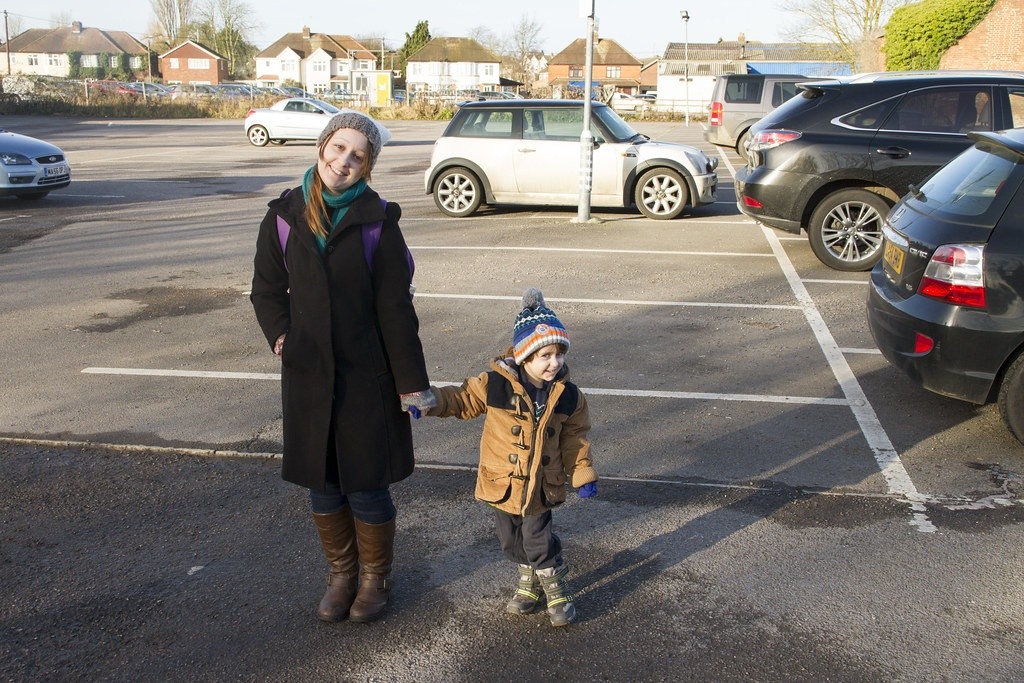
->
[408,404,424,419]
[578,482,598,498]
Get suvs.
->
[729,69,1024,273]
[703,71,853,162]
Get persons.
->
[250,112,436,622]
[408,289,599,627]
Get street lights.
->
[679,9,691,128]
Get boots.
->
[536,559,577,627]
[505,563,545,613]
[308,505,360,622]
[350,506,397,623]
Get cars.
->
[424,98,719,220]
[0,127,71,199]
[865,126,1024,452]
[0,74,351,101]
[394,87,657,111]
[244,97,392,148]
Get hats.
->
[315,108,392,155]
[512,288,572,366]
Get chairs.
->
[973,101,991,131]
[524,114,546,140]
[464,122,486,132]
